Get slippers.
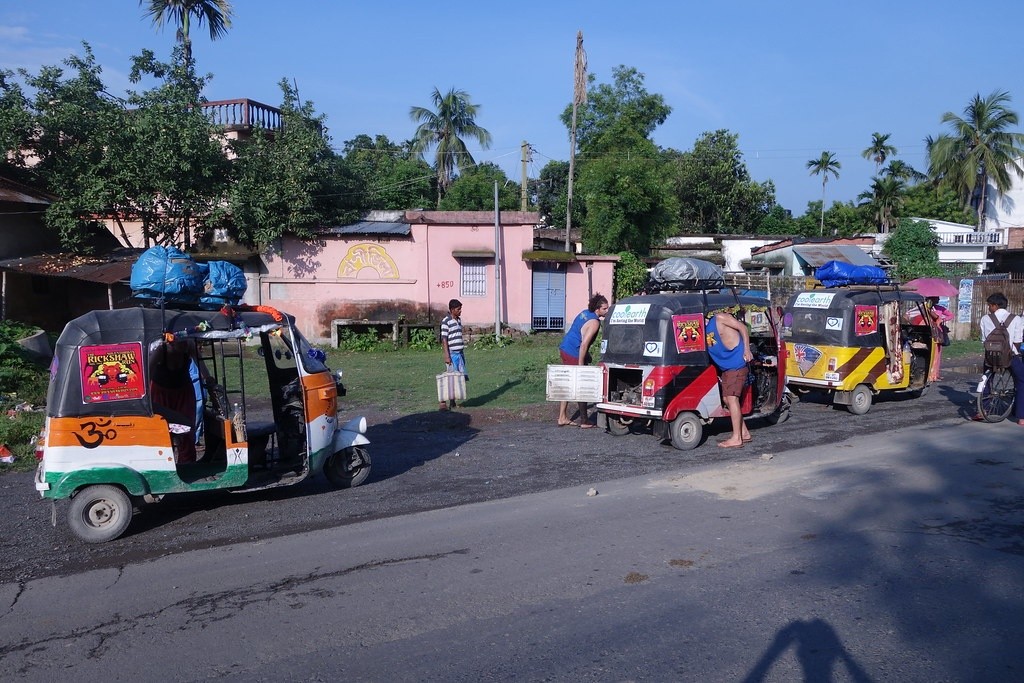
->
[718,437,752,447]
[582,423,597,429]
[561,420,578,426]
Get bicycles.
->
[975,361,1019,424]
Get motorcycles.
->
[27,243,373,546]
[591,255,798,452]
[780,259,941,416]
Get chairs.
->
[212,385,276,470]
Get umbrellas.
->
[902,278,960,307]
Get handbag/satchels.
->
[940,318,951,346]
[437,363,467,402]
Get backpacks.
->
[984,313,1017,368]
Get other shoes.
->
[933,376,944,382]
[974,414,986,420]
[439,405,459,413]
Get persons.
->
[923,296,954,383]
[147,323,217,468]
[703,312,754,448]
[972,292,1024,426]
[439,299,469,413]
[557,293,609,429]
